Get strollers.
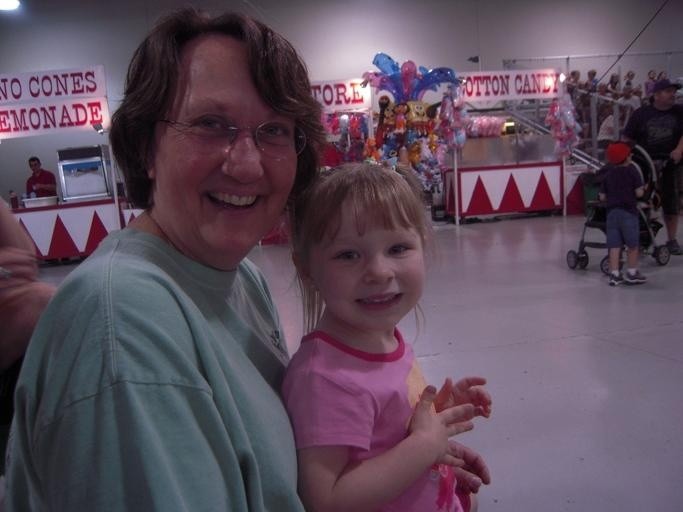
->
[567,140,669,275]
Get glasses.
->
[162,113,309,159]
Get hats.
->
[653,79,683,95]
[608,143,629,165]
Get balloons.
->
[541,97,584,158]
[333,50,508,193]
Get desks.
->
[7,195,127,267]
[442,159,563,225]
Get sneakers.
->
[668,240,683,255]
[610,275,621,286]
[627,272,647,282]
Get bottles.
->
[22,192,37,199]
[9,189,18,209]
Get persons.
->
[624,78,683,256]
[26,156,56,198]
[598,142,649,287]
[564,69,683,162]
[0,197,58,374]
[5,4,329,511]
[280,158,493,511]
